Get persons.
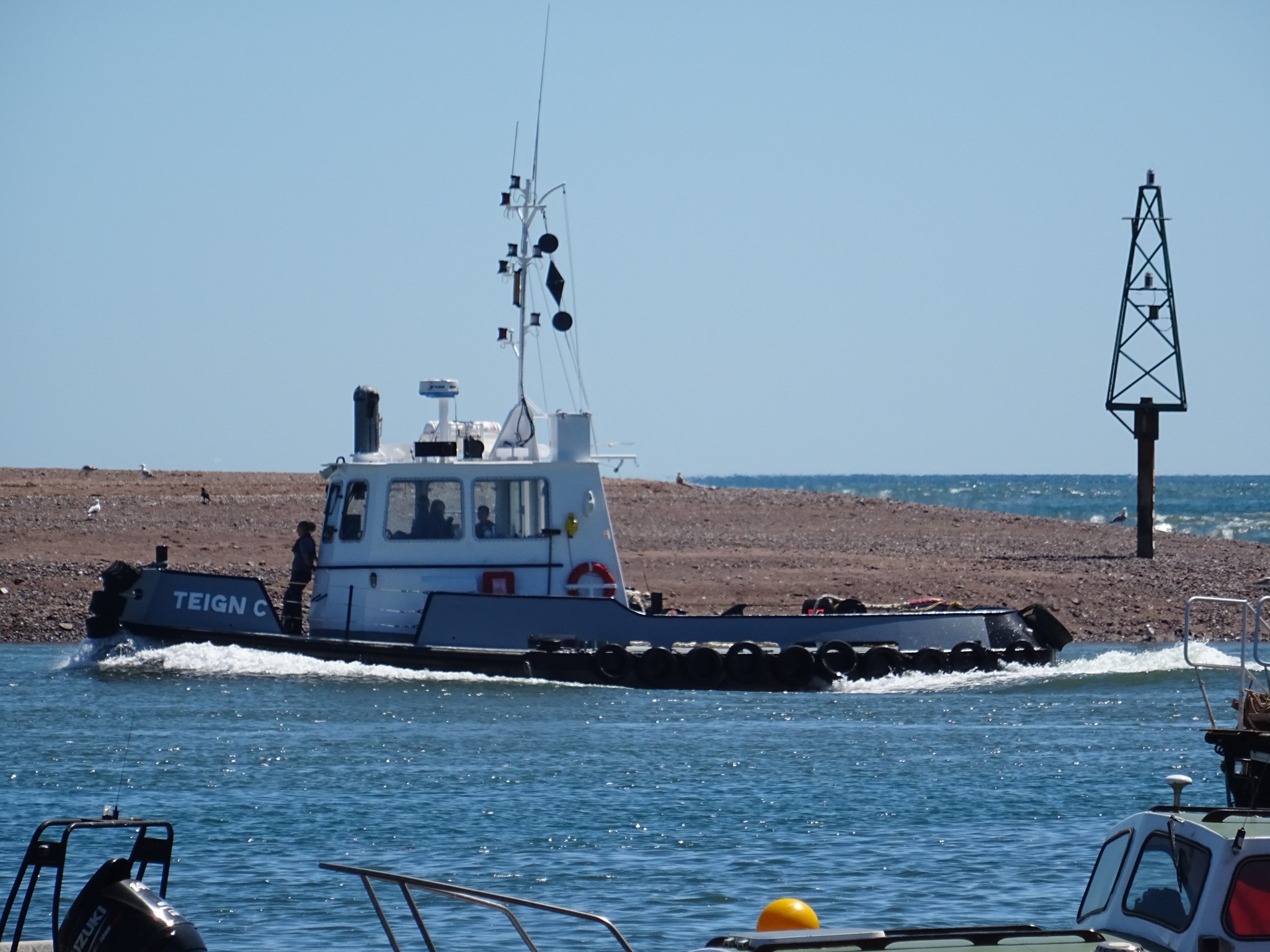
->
[279,520,319,635]
[396,495,453,539]
[474,505,497,539]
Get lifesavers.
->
[913,647,948,674]
[779,645,815,691]
[727,642,764,683]
[566,562,615,597]
[1006,641,1035,666]
[642,647,676,687]
[950,641,991,673]
[817,640,859,681]
[861,647,901,682]
[596,643,634,682]
[685,645,725,687]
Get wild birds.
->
[137,463,157,482]
[675,471,692,488]
[1108,507,1129,527]
[200,486,213,504]
[86,498,101,521]
[78,464,100,477]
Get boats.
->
[81,116,1075,695]
[316,593,1270,952]
